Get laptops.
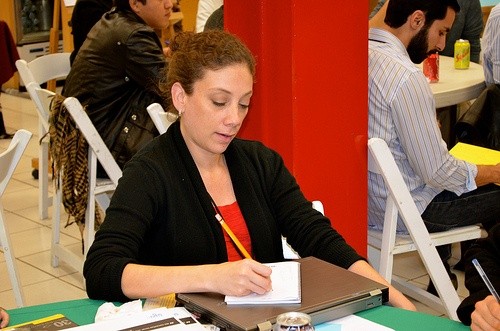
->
[176,256,389,331]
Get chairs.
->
[364,134,489,324]
[28,83,126,284]
[0,127,34,306]
[147,100,181,133]
[15,47,85,215]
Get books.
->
[225,261,301,307]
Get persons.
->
[0,308,9,328]
[368,0,500,298]
[61,0,224,178]
[457,223,500,331]
[0,20,20,139]
[82,29,417,313]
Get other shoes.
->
[426,273,458,297]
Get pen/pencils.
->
[472,259,500,302]
[216,214,273,290]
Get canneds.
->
[271,311,315,331]
[454,38,470,69]
[423,52,439,83]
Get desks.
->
[414,52,486,109]
[0,292,470,331]
